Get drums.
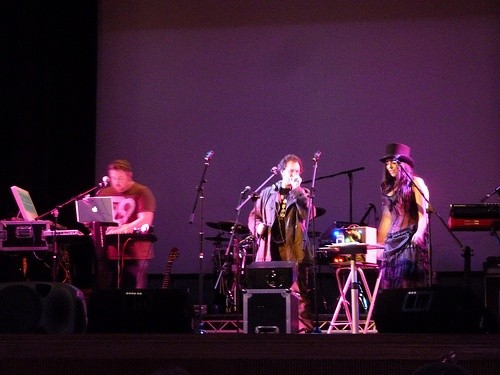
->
[203,236,230,243]
[213,248,254,308]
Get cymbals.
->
[205,220,251,235]
[315,207,327,218]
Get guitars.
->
[163,245,181,289]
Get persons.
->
[248,154,316,334]
[377,142,435,291]
[96,158,155,288]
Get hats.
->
[379,144,414,163]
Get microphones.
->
[294,178,299,182]
[204,151,215,161]
[240,185,251,195]
[271,166,278,175]
[102,175,110,186]
[392,156,399,163]
[313,151,322,162]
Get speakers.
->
[372,286,483,334]
[0,282,195,333]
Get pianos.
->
[445,203,500,273]
[44,226,158,290]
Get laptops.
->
[75,196,119,224]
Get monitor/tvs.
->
[10,186,38,221]
